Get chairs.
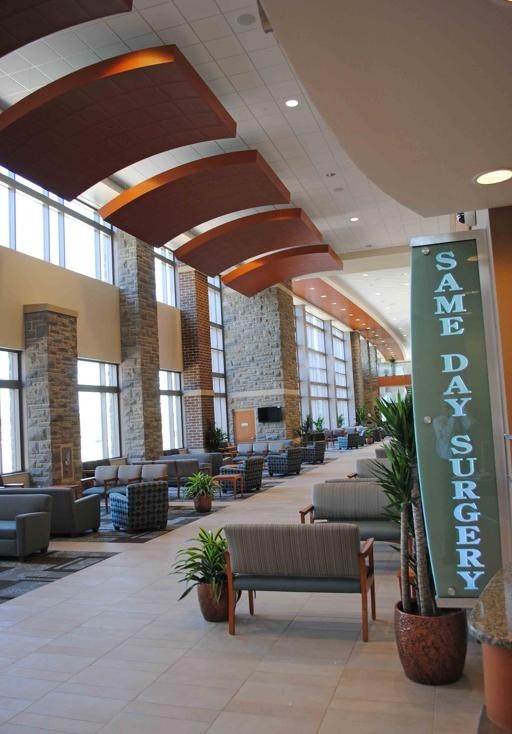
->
[81,441,327,532]
[324,427,366,450]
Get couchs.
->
[0,488,100,565]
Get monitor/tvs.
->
[258,406,281,422]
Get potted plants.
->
[377,387,469,687]
[167,527,242,623]
[366,429,375,445]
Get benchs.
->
[221,457,401,644]
